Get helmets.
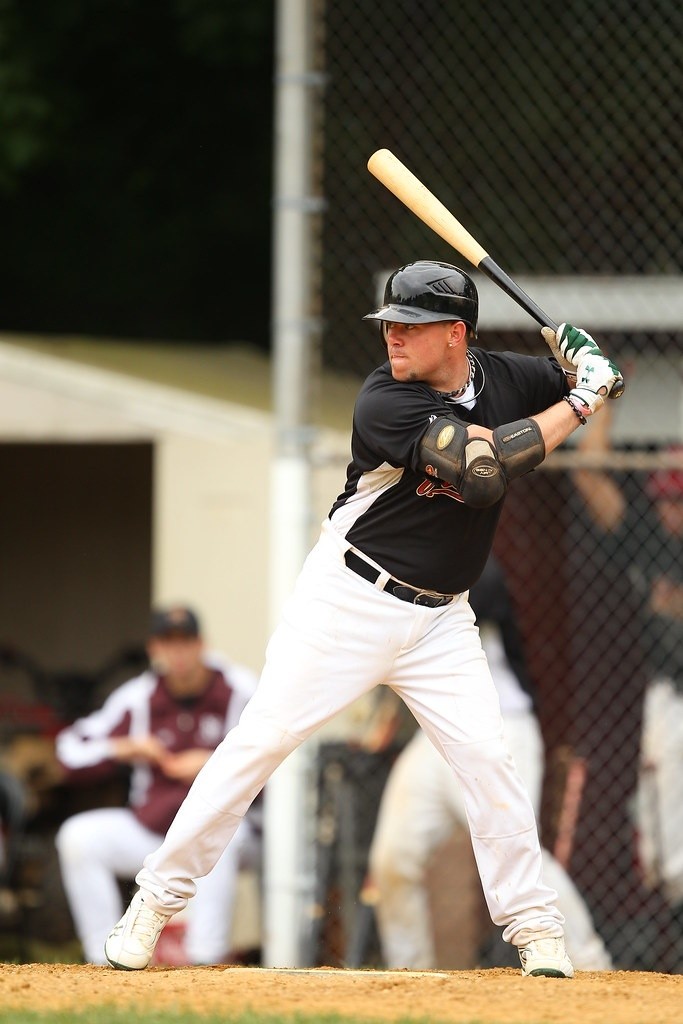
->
[360,260,479,340]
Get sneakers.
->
[105,888,171,971]
[517,935,574,978]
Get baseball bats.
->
[368,146,627,401]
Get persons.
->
[104,260,620,982]
[0,362,683,973]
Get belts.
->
[344,550,453,607]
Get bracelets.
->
[562,393,588,427]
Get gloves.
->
[540,323,602,382]
[569,349,625,414]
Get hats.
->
[146,610,200,642]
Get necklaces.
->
[435,348,485,405]
